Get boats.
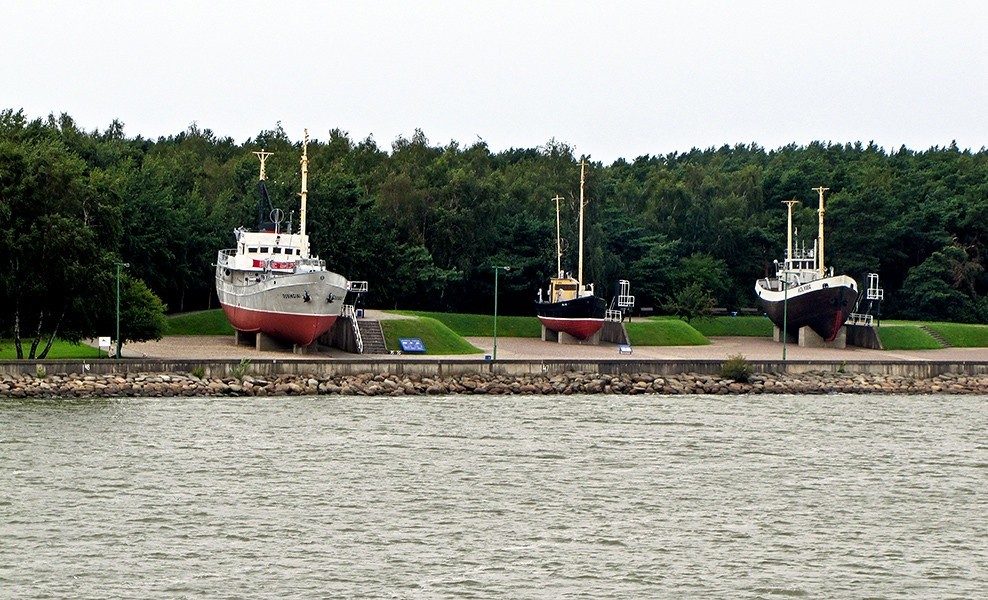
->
[526,161,611,341]
[213,128,370,345]
[753,187,861,343]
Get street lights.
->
[114,261,129,356]
[491,266,510,360]
[778,261,800,366]
